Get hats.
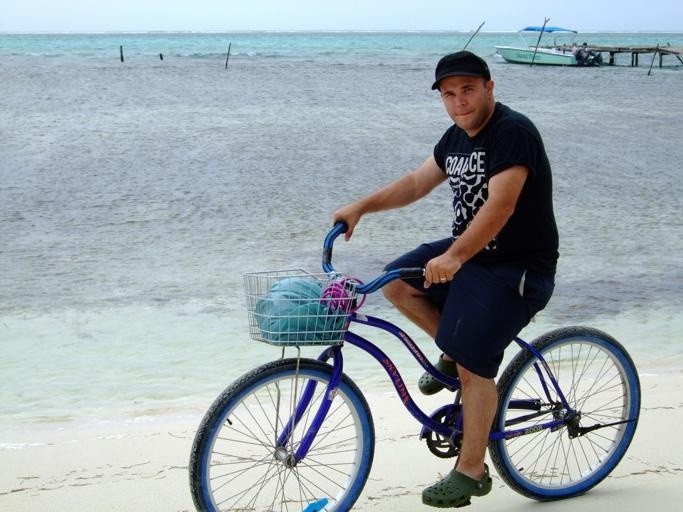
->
[432,51,490,91]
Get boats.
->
[494,45,601,67]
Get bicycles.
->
[188,218,641,512]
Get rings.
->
[440,278,446,281]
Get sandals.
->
[418,354,459,394]
[422,462,492,507]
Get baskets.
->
[240,268,360,346]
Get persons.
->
[332,51,559,508]
